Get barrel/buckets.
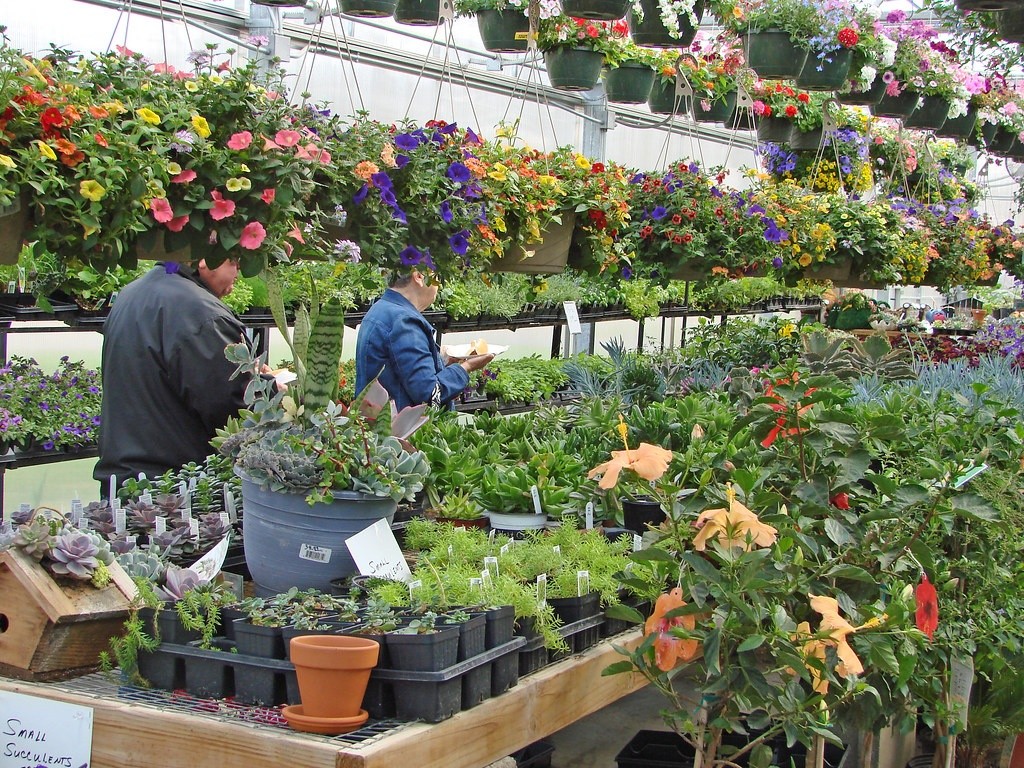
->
[291,636,379,717]
[971,309,986,324]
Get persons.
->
[93,256,282,501]
[355,261,496,413]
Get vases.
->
[626,0,705,48]
[836,73,887,106]
[903,93,949,131]
[796,45,857,92]
[868,82,919,118]
[487,206,577,273]
[693,88,736,122]
[934,102,978,139]
[648,74,697,115]
[540,44,608,92]
[288,637,379,717]
[757,116,791,142]
[803,251,854,280]
[742,28,809,80]
[790,125,823,150]
[600,64,657,104]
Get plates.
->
[281,706,368,731]
[445,341,508,360]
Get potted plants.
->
[0,254,731,691]
[907,654,1024,767]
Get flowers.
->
[583,347,1024,768]
[0,0,1024,458]
